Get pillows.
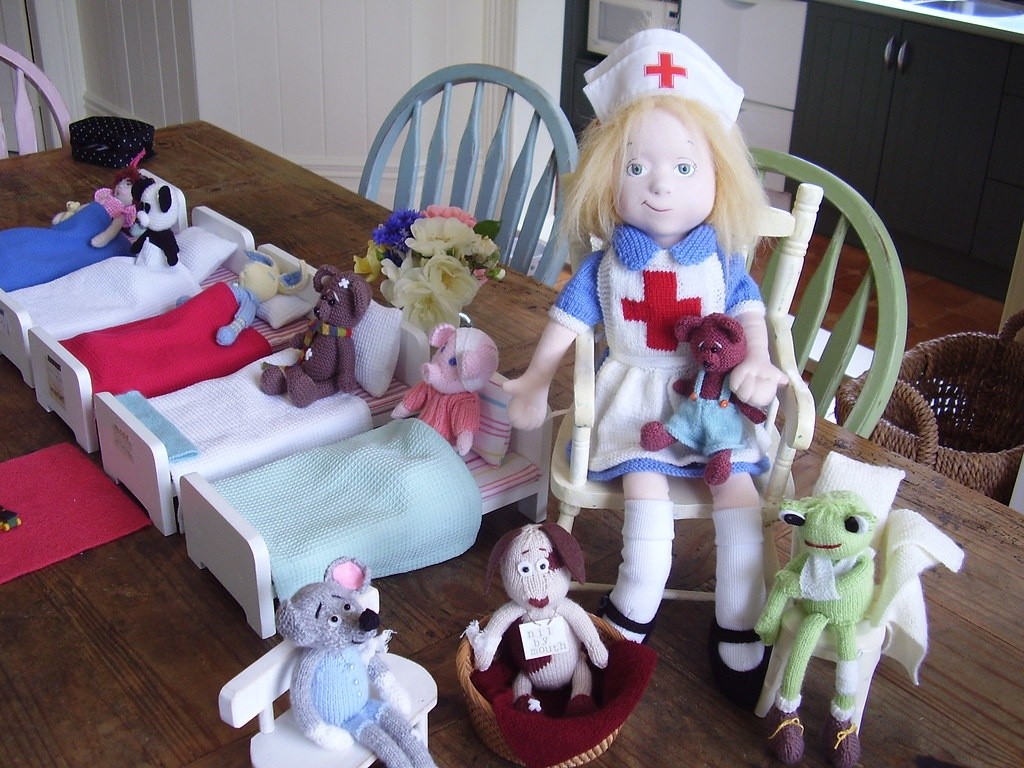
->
[349,298,404,399]
[173,225,237,284]
[809,450,906,555]
[471,379,512,470]
[253,293,314,331]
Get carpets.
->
[0,442,152,586]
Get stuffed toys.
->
[640,313,766,488]
[51,165,142,248]
[754,492,874,765]
[390,323,499,456]
[260,265,372,407]
[459,523,607,715]
[130,181,180,265]
[216,248,308,345]
[275,556,438,768]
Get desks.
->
[0,120,1024,768]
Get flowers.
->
[349,202,508,336]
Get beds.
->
[0,166,553,642]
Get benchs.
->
[217,637,439,768]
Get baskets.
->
[456,612,630,767]
[834,310,1024,507]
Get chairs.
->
[356,61,584,290]
[755,524,887,741]
[744,145,908,441]
[0,40,74,163]
[548,180,829,607]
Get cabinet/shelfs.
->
[554,0,1024,303]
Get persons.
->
[502,28,790,707]
